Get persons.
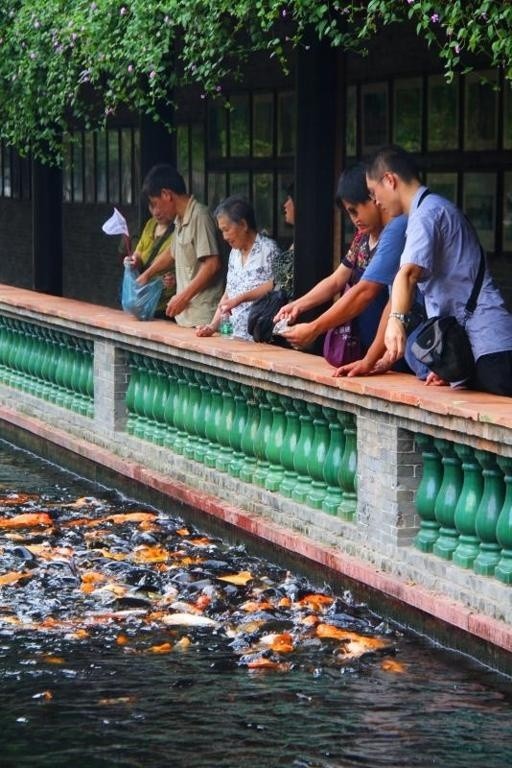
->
[123,165,294,343]
[272,145,512,397]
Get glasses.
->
[347,205,359,218]
[368,176,385,202]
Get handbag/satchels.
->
[410,314,475,382]
[323,258,361,368]
[248,291,288,343]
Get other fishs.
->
[0,485,410,676]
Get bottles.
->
[220,305,232,339]
